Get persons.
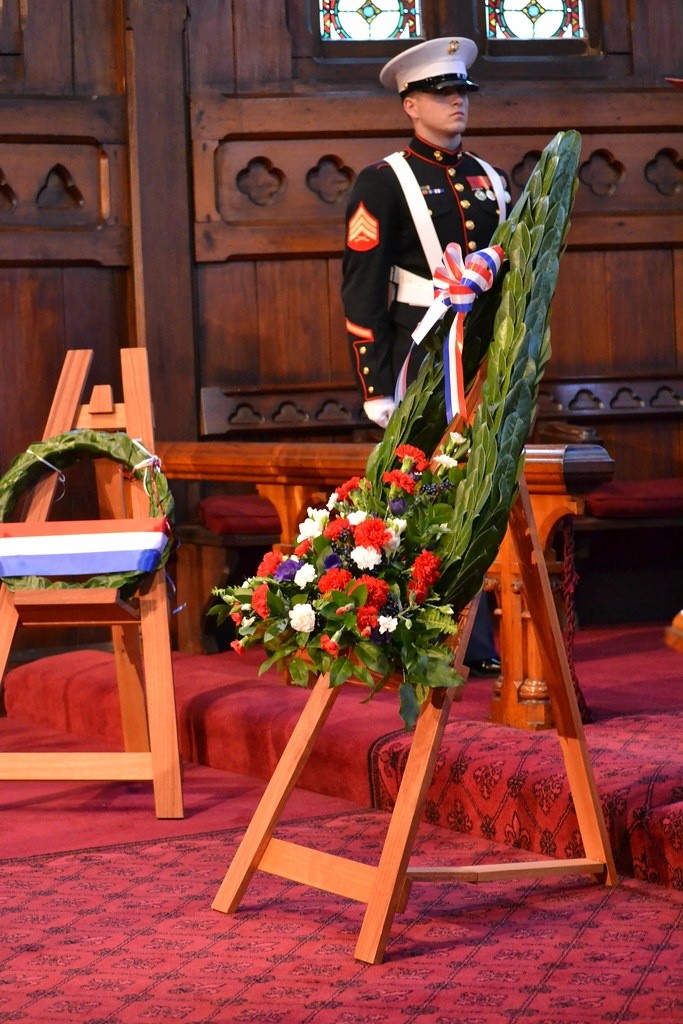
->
[342,37,512,679]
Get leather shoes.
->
[464,657,501,677]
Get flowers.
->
[210,118,584,734]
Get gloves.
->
[364,395,397,428]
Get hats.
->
[380,37,480,97]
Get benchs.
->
[173,475,683,545]
